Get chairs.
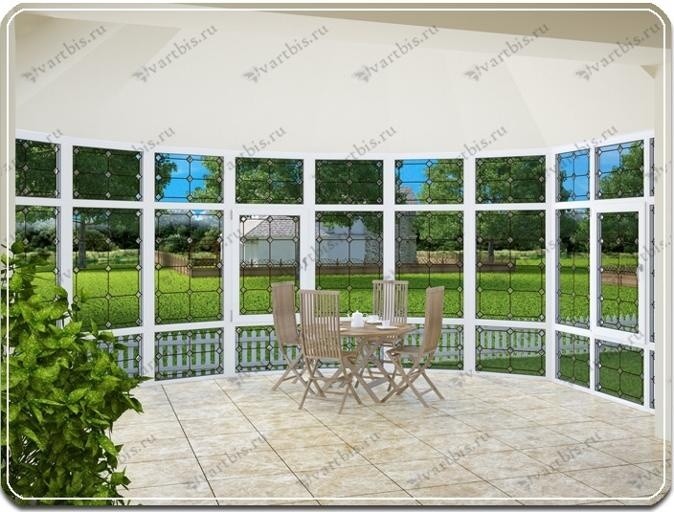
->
[357,280,409,392]
[298,290,361,414]
[270,280,332,396]
[380,285,445,407]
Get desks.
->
[300,320,419,403]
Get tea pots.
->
[346,309,368,329]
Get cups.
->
[381,320,390,327]
[370,315,379,322]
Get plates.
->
[366,320,382,324]
[376,325,399,330]
[329,328,349,331]
